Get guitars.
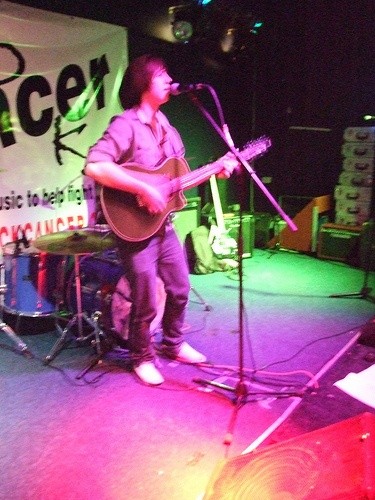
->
[100,135,271,246]
[208,174,238,259]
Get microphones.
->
[22,229,30,248]
[170,82,207,96]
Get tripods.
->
[329,227,374,301]
[188,93,304,445]
[0,232,131,379]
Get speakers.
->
[204,411,375,500]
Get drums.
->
[68,249,166,341]
[3,240,67,316]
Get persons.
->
[84,53,238,385]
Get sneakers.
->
[128,362,165,385]
[165,341,207,364]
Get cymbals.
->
[33,230,118,252]
